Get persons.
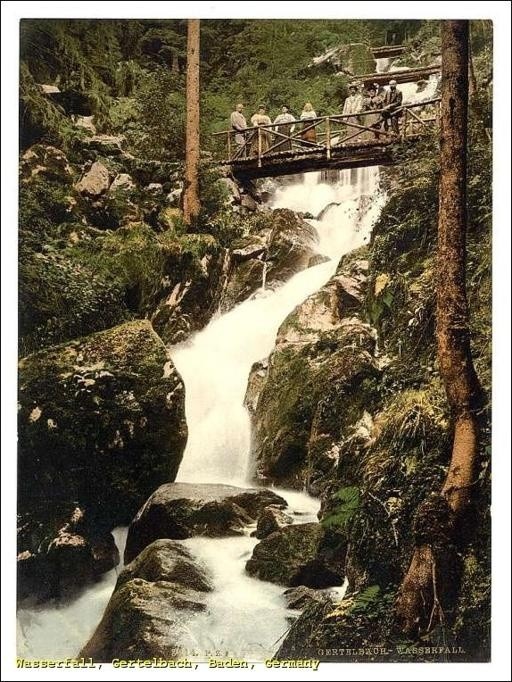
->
[231,103,247,157]
[273,105,296,153]
[341,80,402,139]
[249,105,272,157]
[300,102,317,147]
